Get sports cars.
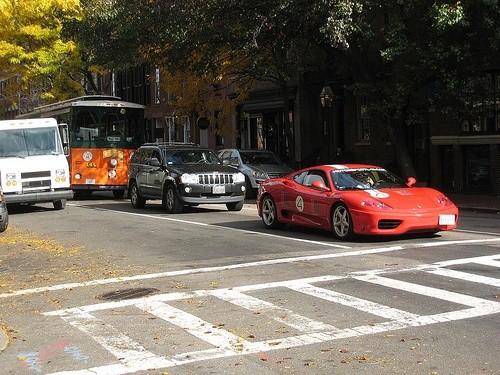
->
[256,164,458,240]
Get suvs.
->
[127,142,246,214]
[217,148,295,200]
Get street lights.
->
[320,86,335,164]
[172,112,179,142]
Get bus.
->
[14,95,147,200]
[0,117,73,210]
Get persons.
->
[357,173,374,189]
[110,124,124,141]
[246,154,256,163]
